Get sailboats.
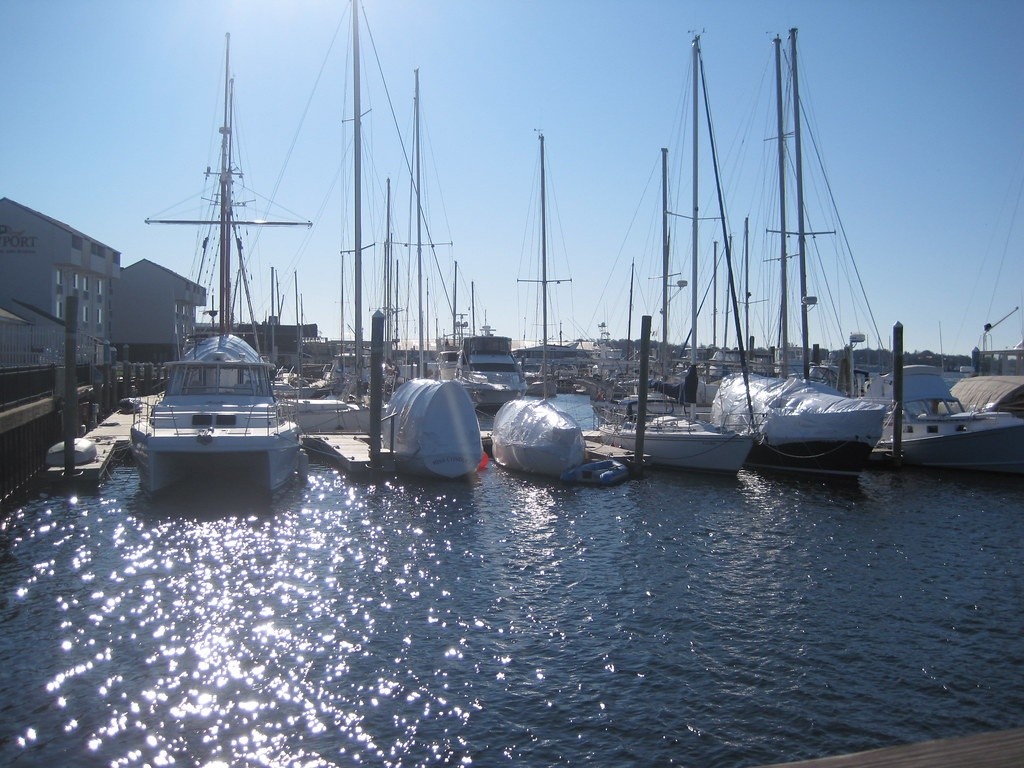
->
[713,34,887,477]
[948,374,1024,419]
[123,37,310,501]
[303,0,489,487]
[580,26,764,474]
[194,70,951,396]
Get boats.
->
[857,365,1024,479]
[478,394,653,486]
